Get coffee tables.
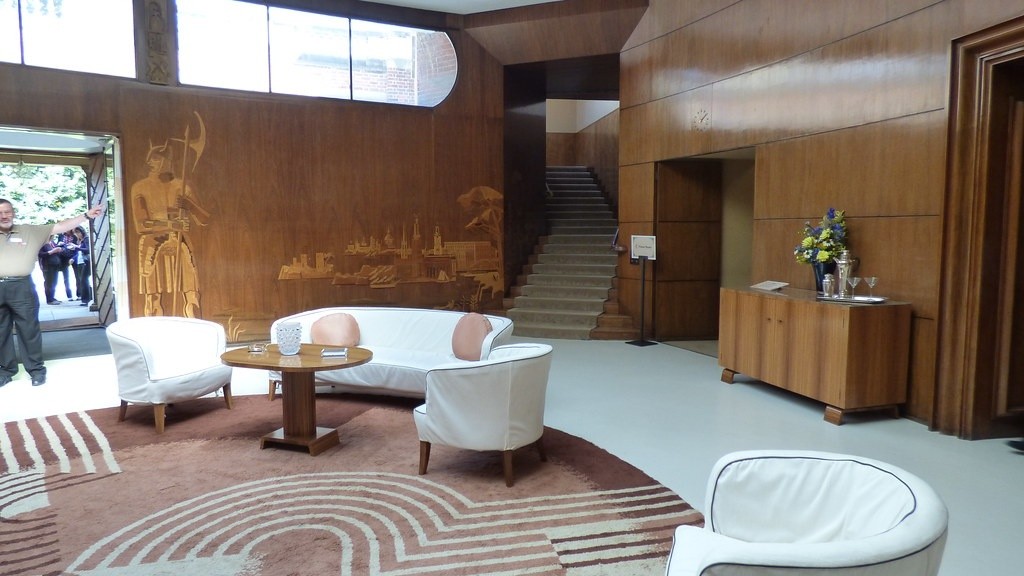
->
[219,344,374,457]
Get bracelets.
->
[84,213,92,221]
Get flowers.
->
[794,208,849,264]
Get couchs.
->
[106,316,235,434]
[413,342,554,486]
[666,450,949,576]
[268,306,514,401]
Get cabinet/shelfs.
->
[717,286,912,426]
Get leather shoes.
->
[0,378,12,387]
[31,375,46,387]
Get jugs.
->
[832,249,860,298]
[823,273,835,296]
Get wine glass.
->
[845,277,861,300]
[865,275,879,300]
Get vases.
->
[811,261,837,294]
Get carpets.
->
[2,393,705,576]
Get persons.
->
[68,225,90,307]
[0,198,104,387]
[37,233,62,305]
[132,110,205,322]
[54,227,87,302]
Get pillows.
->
[311,313,360,347]
[452,313,493,361]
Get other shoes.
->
[48,296,91,308]
